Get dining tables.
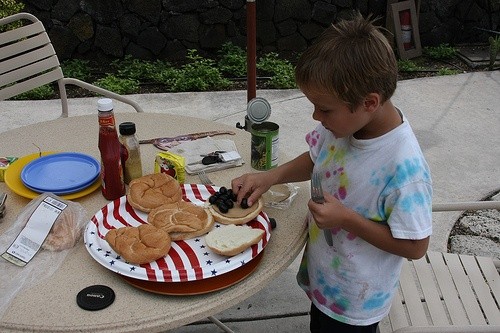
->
[0,112,311,332]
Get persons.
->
[231,10,433,333]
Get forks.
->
[198,171,215,186]
[310,173,333,246]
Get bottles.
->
[97,98,126,201]
[119,122,142,185]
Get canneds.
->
[247,97,280,171]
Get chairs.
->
[1,11,144,119]
[376,201,500,333]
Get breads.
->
[127,172,182,213]
[105,224,171,264]
[205,224,265,256]
[208,191,264,225]
[147,202,215,240]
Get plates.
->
[84,184,271,282]
[4,151,102,199]
